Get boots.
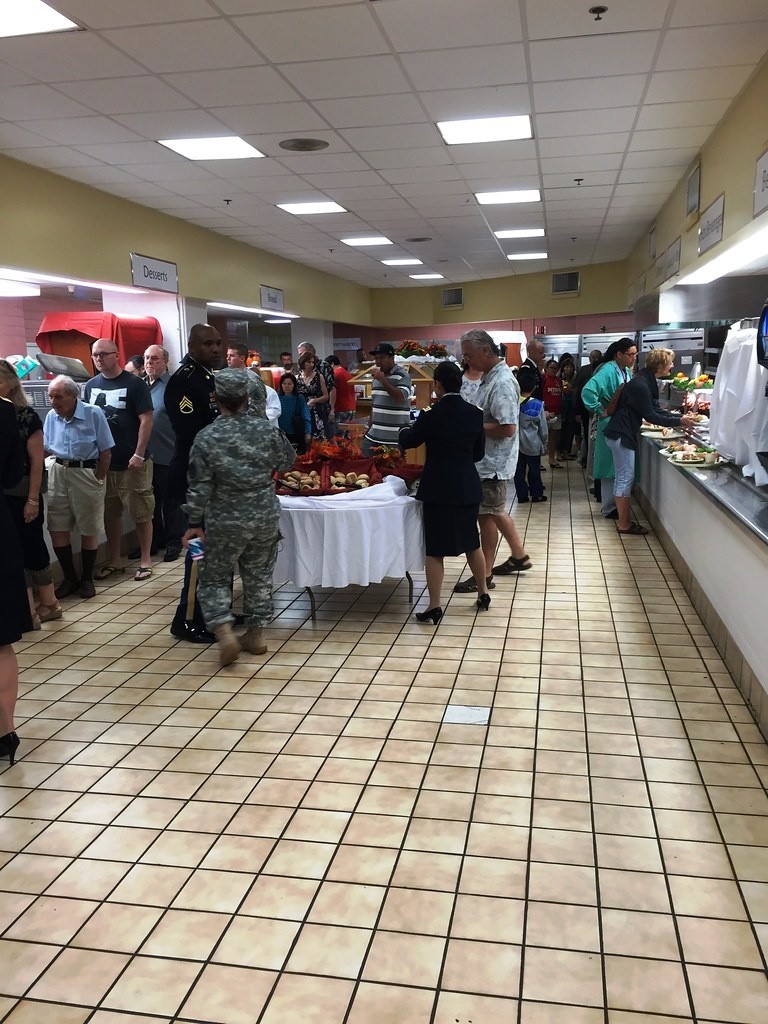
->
[52,545,79,598]
[214,623,241,667]
[79,548,98,597]
[240,628,267,654]
[134,566,153,581]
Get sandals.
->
[454,573,495,593]
[31,600,62,629]
[492,555,532,574]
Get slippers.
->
[550,463,563,468]
[94,566,126,580]
[540,465,546,471]
[616,521,648,534]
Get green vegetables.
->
[696,447,715,454]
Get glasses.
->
[624,352,638,357]
[547,366,559,370]
[461,344,484,360]
[91,352,117,360]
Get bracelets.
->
[134,453,145,461]
[27,498,40,507]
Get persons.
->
[0,322,700,766]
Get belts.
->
[55,457,97,468]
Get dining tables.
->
[276,495,423,623]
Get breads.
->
[283,470,370,490]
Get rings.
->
[31,517,34,520]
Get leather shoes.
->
[168,613,246,642]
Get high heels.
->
[0,732,20,766]
[476,593,491,612]
[416,606,442,626]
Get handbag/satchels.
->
[315,400,332,421]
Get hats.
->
[368,343,395,357]
[214,368,249,398]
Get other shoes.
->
[163,549,179,562]
[556,454,574,462]
[531,495,547,502]
[604,509,618,519]
[517,498,529,503]
[128,548,158,559]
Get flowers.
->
[395,337,447,356]
[677,372,708,381]
[311,434,402,465]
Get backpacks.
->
[606,376,648,417]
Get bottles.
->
[691,362,701,379]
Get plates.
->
[637,412,718,466]
